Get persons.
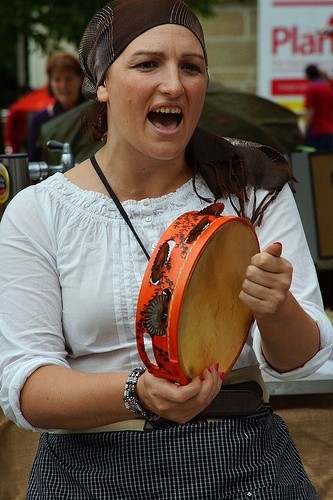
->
[26,51,89,162]
[302,64,333,152]
[0,0,333,500]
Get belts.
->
[192,381,265,419]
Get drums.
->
[132,203,263,389]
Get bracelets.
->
[123,367,161,422]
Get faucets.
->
[46,138,74,172]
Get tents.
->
[6,85,54,155]
[38,76,305,161]
